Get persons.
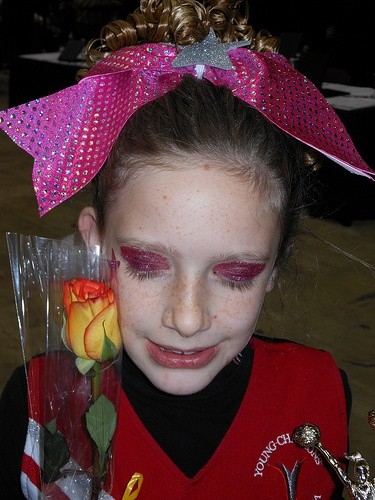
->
[0,0,375,499]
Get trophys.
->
[282,410,375,500]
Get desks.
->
[7,45,375,226]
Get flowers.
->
[59,278,122,375]
